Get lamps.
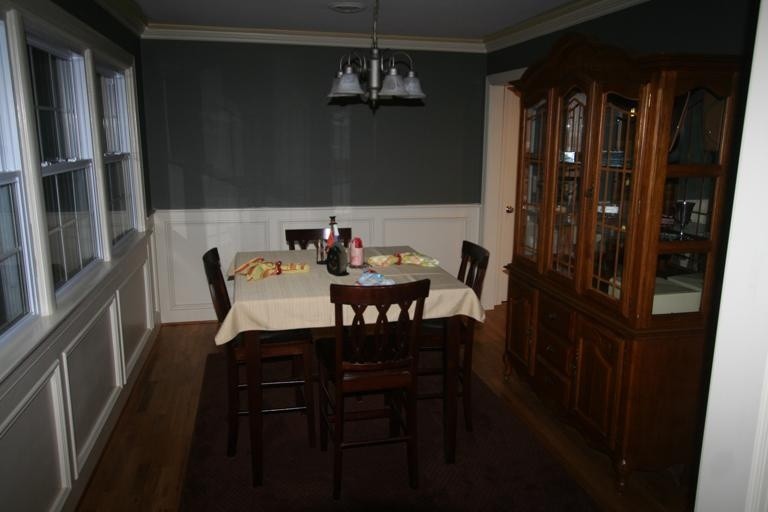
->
[327,0,427,114]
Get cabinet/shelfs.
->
[503,264,714,493]
[507,46,746,335]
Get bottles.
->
[349,234,362,268]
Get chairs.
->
[318,280,433,500]
[284,227,354,250]
[202,246,326,459]
[372,238,491,465]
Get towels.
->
[355,269,395,286]
[368,252,438,268]
[236,257,309,281]
